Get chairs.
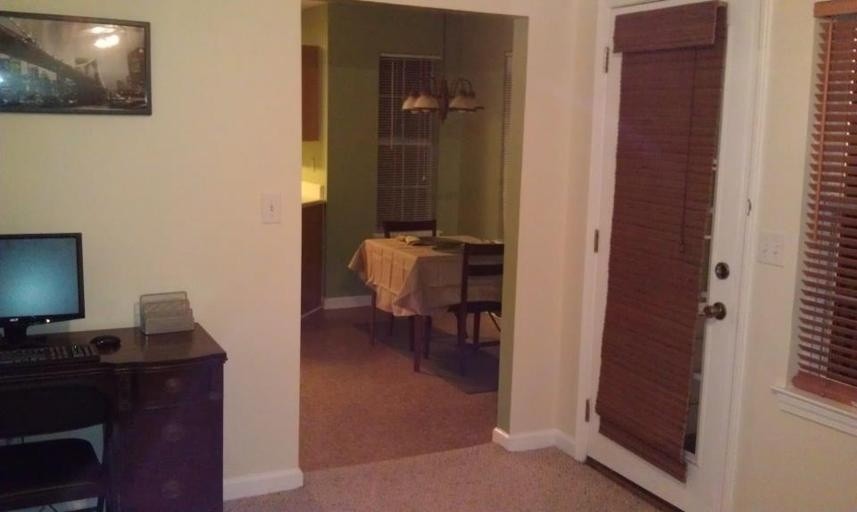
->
[447,242,504,377]
[0,386,113,512]
[381,219,437,352]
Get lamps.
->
[401,9,485,124]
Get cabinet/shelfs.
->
[302,202,325,319]
[302,45,318,142]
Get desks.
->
[0,322,227,512]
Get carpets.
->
[222,441,663,511]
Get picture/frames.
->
[0,10,152,116]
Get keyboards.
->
[0,341,102,371]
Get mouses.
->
[90,333,121,349]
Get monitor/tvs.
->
[0,232,86,346]
